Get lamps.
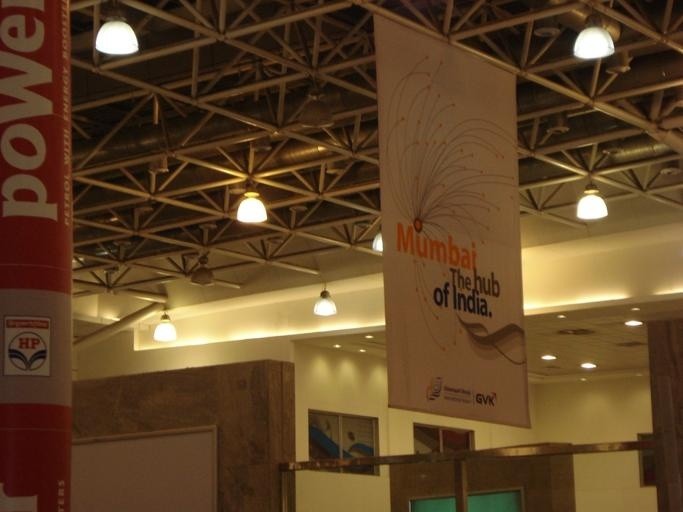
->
[313,285,338,317]
[573,5,616,59]
[372,230,383,254]
[237,185,267,223]
[152,311,178,344]
[93,1,141,57]
[576,179,609,221]
